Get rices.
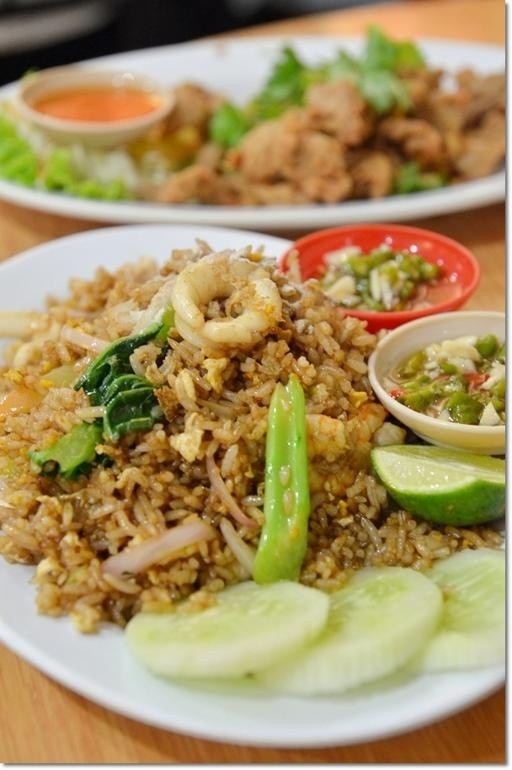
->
[0,239,505,635]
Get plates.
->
[12,61,176,153]
[1,222,505,752]
[1,32,505,232]
[282,222,480,335]
[364,309,505,457]
